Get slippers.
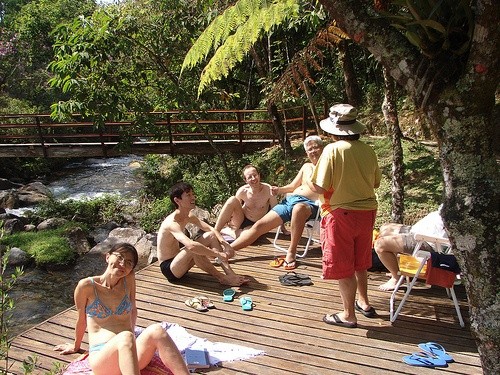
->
[197,294,216,308]
[284,260,301,270]
[402,351,449,367]
[185,298,207,310]
[323,312,357,328]
[354,299,375,317]
[240,296,252,310]
[223,288,235,301]
[280,276,313,286]
[279,271,311,281]
[417,342,454,363]
[270,255,286,268]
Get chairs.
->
[274,202,322,258]
[390,231,466,328]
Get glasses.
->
[112,252,138,268]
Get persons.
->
[229,135,324,269]
[214,163,292,241]
[310,104,382,327]
[53,242,191,375]
[157,182,250,286]
[373,200,453,291]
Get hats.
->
[319,103,366,136]
